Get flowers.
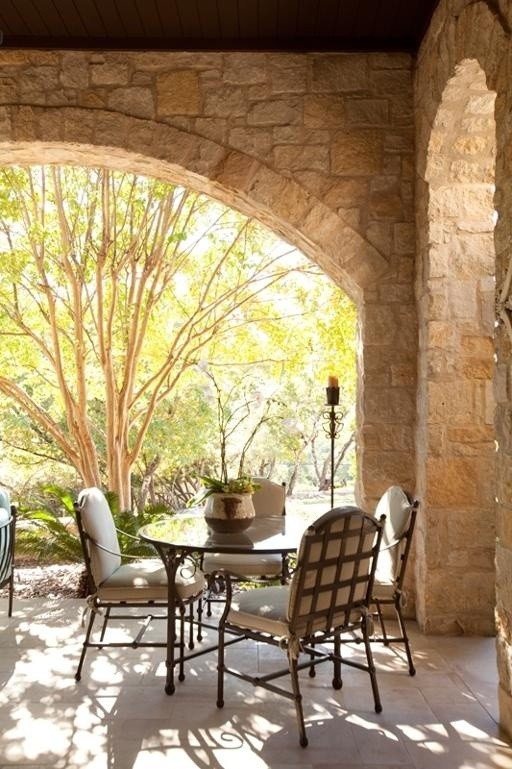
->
[186,475,263,509]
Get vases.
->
[204,492,256,534]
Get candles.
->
[325,376,338,387]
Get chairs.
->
[206,477,286,617]
[74,486,204,682]
[309,484,418,678]
[217,505,386,747]
[0,492,18,618]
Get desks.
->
[137,513,308,695]
